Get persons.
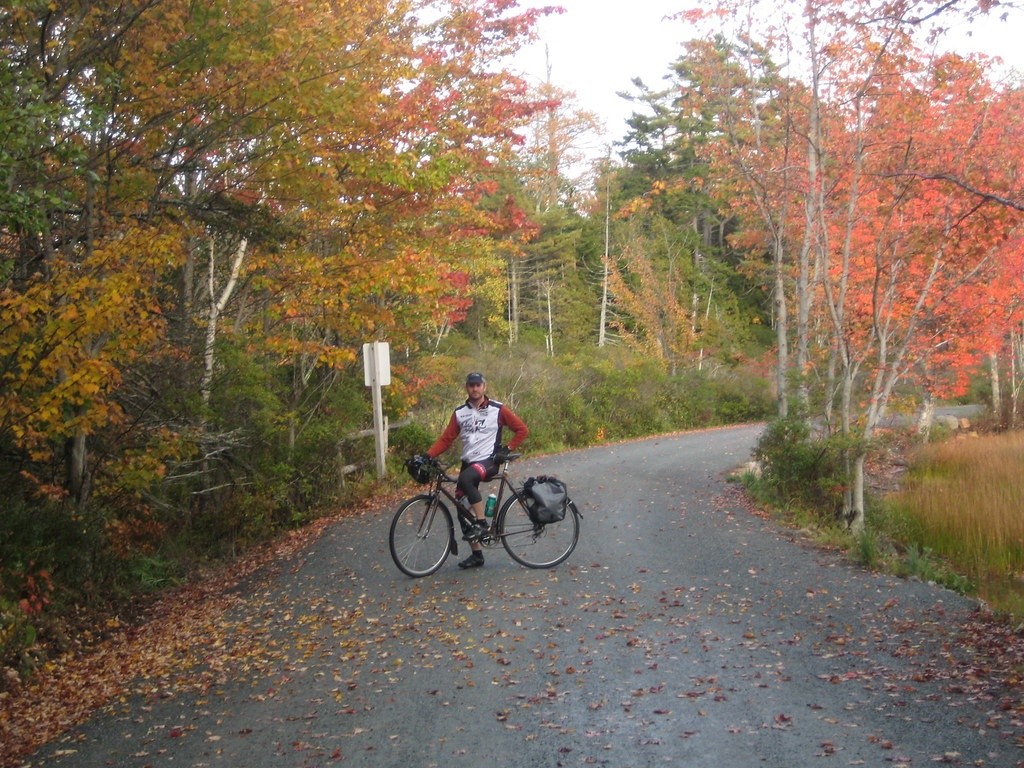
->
[426,372,529,569]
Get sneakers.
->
[458,554,484,567]
[462,521,491,541]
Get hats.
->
[466,372,485,383]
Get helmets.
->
[403,459,430,484]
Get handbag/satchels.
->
[528,479,568,523]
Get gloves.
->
[414,453,431,465]
[491,446,512,465]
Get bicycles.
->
[389,453,586,578]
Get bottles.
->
[459,496,476,516]
[484,486,498,517]
